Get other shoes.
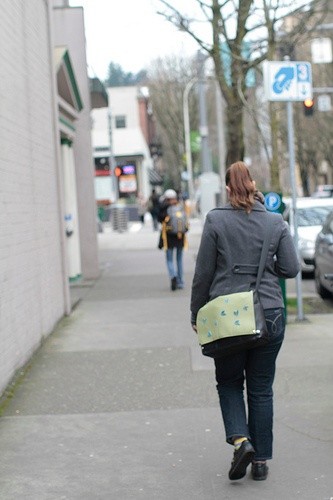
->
[252,460,268,480]
[171,276,176,291]
[229,440,255,480]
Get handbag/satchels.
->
[196,291,269,357]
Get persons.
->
[157,189,188,291]
[148,189,160,231]
[190,161,300,481]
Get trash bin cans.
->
[111,205,129,232]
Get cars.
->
[314,209,333,300]
[279,196,333,274]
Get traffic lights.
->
[303,99,314,116]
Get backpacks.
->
[162,204,189,251]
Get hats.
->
[164,189,178,200]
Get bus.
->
[94,148,149,221]
